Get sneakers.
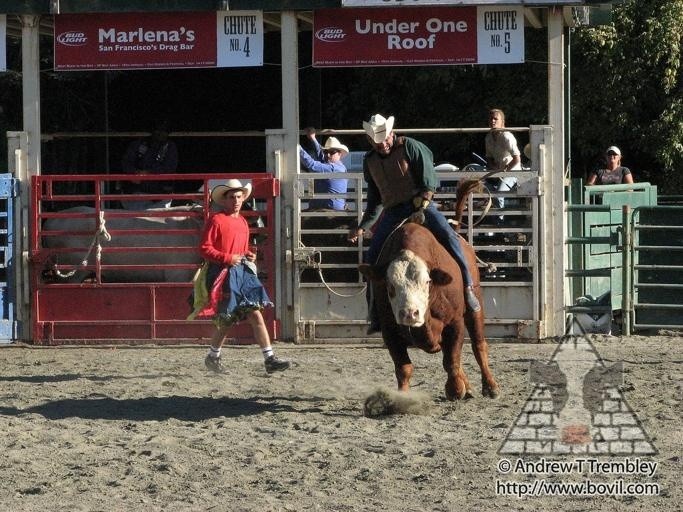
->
[205,353,230,373]
[264,354,289,372]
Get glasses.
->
[322,150,340,155]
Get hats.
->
[211,180,252,204]
[607,145,621,155]
[363,113,395,142]
[321,135,349,157]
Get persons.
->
[480,108,523,236]
[345,115,481,338]
[297,128,353,247]
[187,177,292,377]
[579,144,636,205]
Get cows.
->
[43,198,268,283]
[367,178,500,402]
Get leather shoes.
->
[464,286,481,313]
